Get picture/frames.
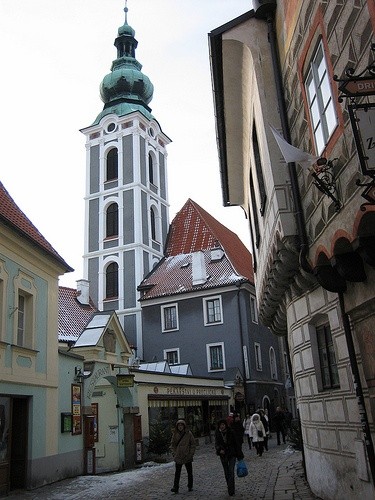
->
[61,383,82,435]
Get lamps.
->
[74,366,84,384]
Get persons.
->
[215,418,245,497]
[168,418,197,493]
[226,407,293,457]
